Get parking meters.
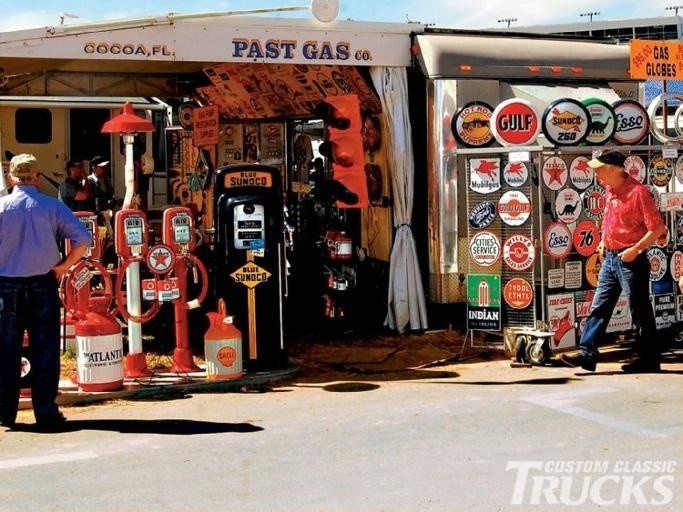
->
[115,208,149,258]
[65,211,98,259]
[163,206,196,254]
[226,196,269,250]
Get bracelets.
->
[632,245,642,255]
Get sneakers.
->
[621,358,662,373]
[560,351,598,371]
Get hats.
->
[8,153,39,177]
[586,147,627,170]
[90,155,110,167]
[65,158,84,167]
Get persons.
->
[0,151,93,427]
[310,157,324,180]
[559,151,667,372]
[0,148,115,226]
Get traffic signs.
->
[629,39,683,80]
